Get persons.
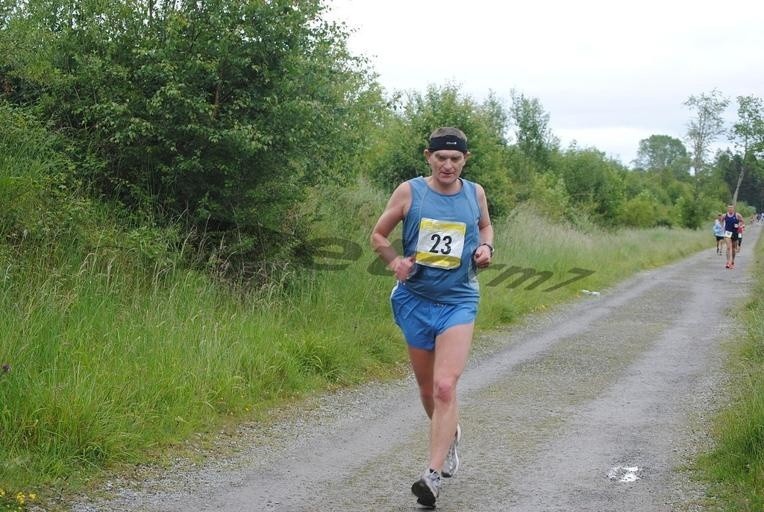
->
[720,204,746,269]
[713,213,725,255]
[368,126,496,512]
[736,222,745,252]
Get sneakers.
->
[441,423,462,478]
[411,469,440,507]
[725,262,734,268]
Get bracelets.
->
[480,243,494,259]
